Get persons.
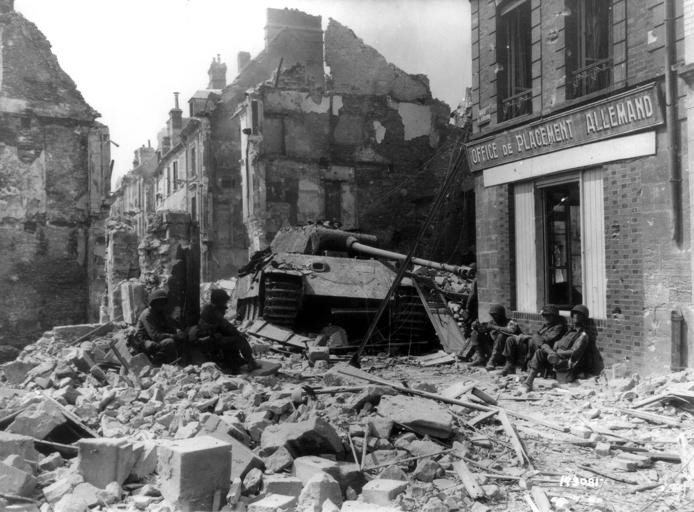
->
[518,303,588,392]
[465,303,522,371]
[184,288,262,375]
[131,288,188,366]
[498,303,567,375]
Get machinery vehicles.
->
[228,224,477,354]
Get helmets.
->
[540,305,560,316]
[571,305,589,318]
[148,291,169,304]
[489,305,507,315]
